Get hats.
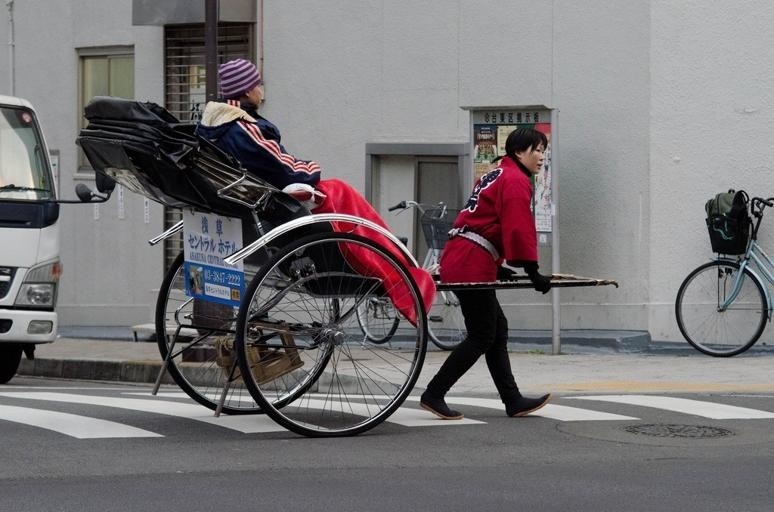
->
[215,56,262,98]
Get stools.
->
[214,328,304,387]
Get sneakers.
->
[503,388,552,417]
[420,387,464,420]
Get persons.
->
[193,58,436,327]
[419,127,554,420]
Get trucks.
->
[0,95,116,384]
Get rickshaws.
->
[75,96,618,438]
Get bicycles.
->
[675,197,774,357]
[355,200,467,350]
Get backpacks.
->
[704,188,750,256]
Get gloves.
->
[496,264,518,282]
[525,262,552,295]
